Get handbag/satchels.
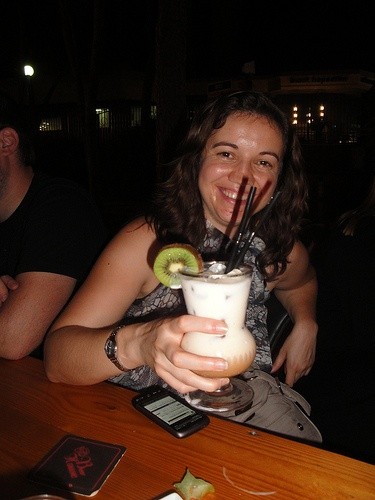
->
[222,367,322,446]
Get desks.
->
[0,356,374,500]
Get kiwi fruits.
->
[152,244,204,290]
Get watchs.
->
[104,325,136,372]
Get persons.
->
[44,91,326,450]
[0,92,114,360]
[336,175,375,237]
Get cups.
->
[180,261,256,412]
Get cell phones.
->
[133,386,210,438]
